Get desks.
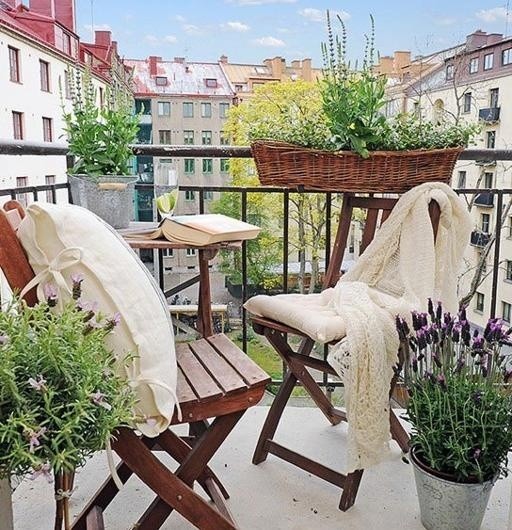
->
[124,231,243,337]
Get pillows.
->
[12,203,179,435]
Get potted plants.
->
[220,10,493,193]
[57,46,146,230]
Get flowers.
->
[390,293,511,479]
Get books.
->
[121,214,263,247]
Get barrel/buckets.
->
[67,172,140,228]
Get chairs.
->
[250,190,443,515]
[0,199,270,528]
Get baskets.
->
[250,141,465,193]
